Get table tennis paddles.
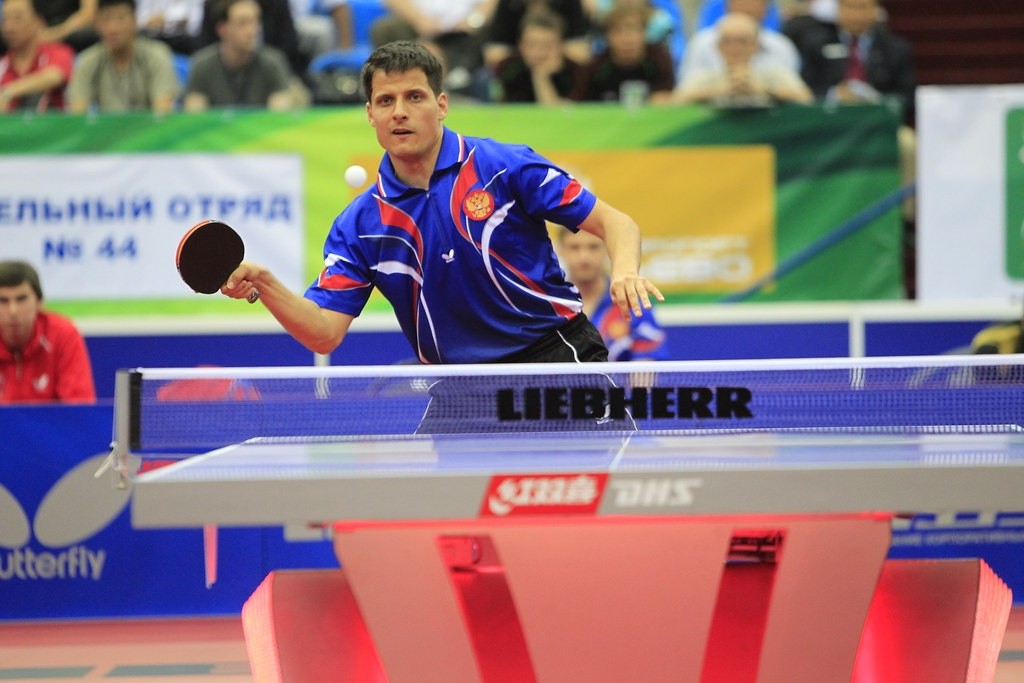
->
[176,219,259,304]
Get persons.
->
[557,223,667,389]
[0,260,97,405]
[0,0,923,220]
[221,41,668,438]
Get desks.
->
[110,349,1024,683]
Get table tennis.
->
[345,165,368,188]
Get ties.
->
[848,35,866,82]
